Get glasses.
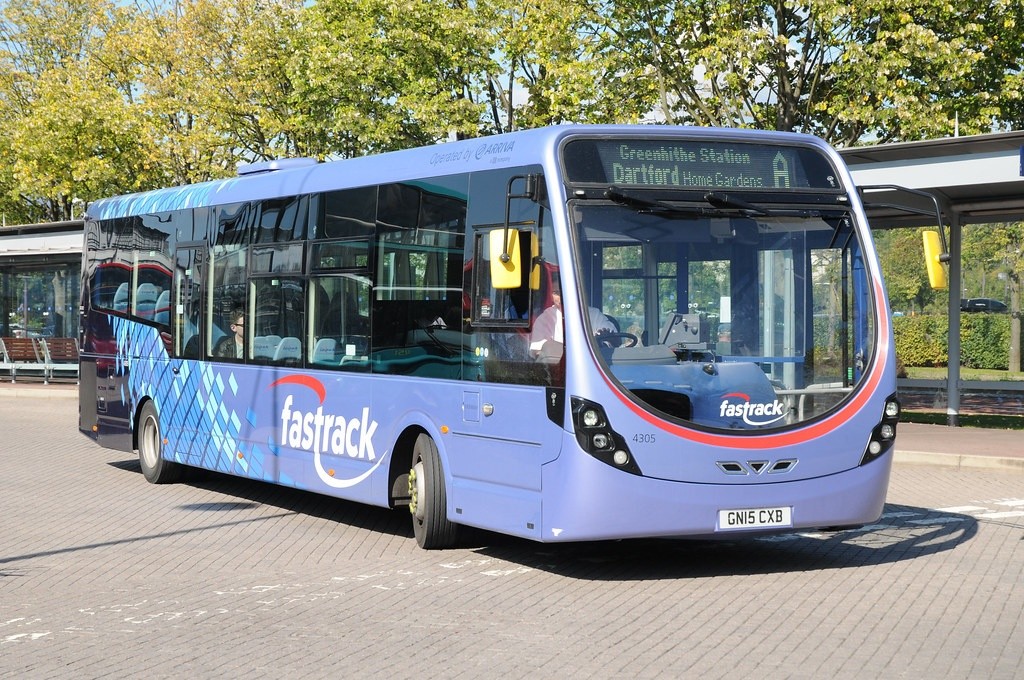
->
[234,323,243,327]
[552,289,560,295]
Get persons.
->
[216,306,244,360]
[528,271,622,364]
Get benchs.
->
[0,339,43,383]
[39,337,80,385]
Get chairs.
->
[113,283,370,369]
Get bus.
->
[79,123,950,553]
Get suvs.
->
[960,298,1009,315]
